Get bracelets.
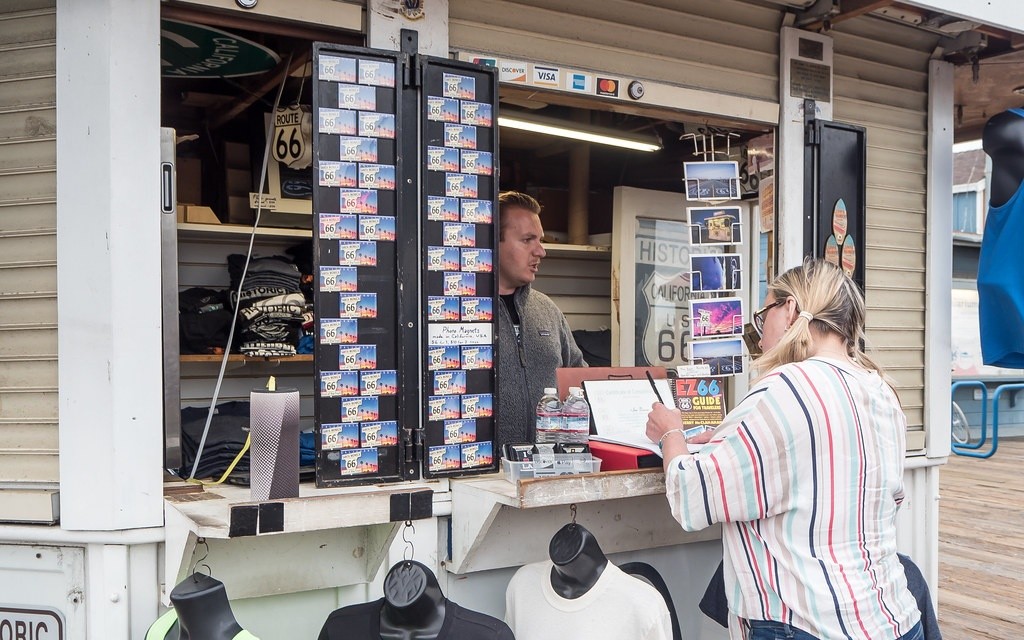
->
[659,429,685,451]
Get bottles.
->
[562,387,589,443]
[536,388,562,442]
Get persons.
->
[643,257,925,640]
[144,573,259,640]
[503,521,674,640]
[498,189,590,457]
[318,559,516,640]
[978,107,1024,368]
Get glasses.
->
[754,299,786,334]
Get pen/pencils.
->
[646,370,665,406]
